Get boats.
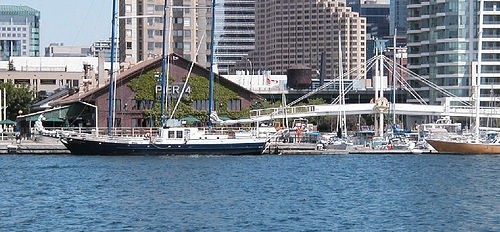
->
[424,86,500,154]
[262,83,348,157]
[54,126,268,155]
[388,139,410,150]
